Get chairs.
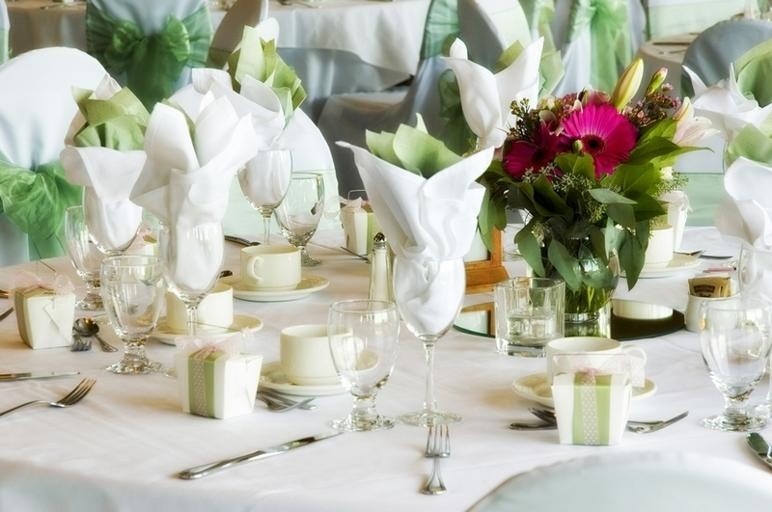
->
[526,2,770,107]
[314,2,530,195]
[223,105,343,227]
[2,46,119,266]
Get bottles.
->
[368,233,391,306]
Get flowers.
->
[476,57,713,291]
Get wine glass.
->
[58,188,166,374]
[159,216,225,381]
[393,240,466,427]
[696,298,771,431]
[328,297,399,435]
[237,148,292,245]
[274,172,323,265]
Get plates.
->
[512,375,656,405]
[152,313,264,348]
[219,276,332,302]
[258,361,356,396]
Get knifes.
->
[181,428,342,478]
[0,371,80,381]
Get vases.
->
[532,260,611,339]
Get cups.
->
[494,274,567,359]
[546,335,645,390]
[279,324,356,384]
[499,223,534,278]
[239,244,304,292]
[169,285,234,334]
[612,192,772,330]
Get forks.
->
[0,377,99,418]
[512,409,690,433]
[257,382,317,414]
[422,420,453,492]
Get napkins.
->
[683,40,768,249]
[439,35,543,146]
[334,111,497,339]
[61,19,307,300]
